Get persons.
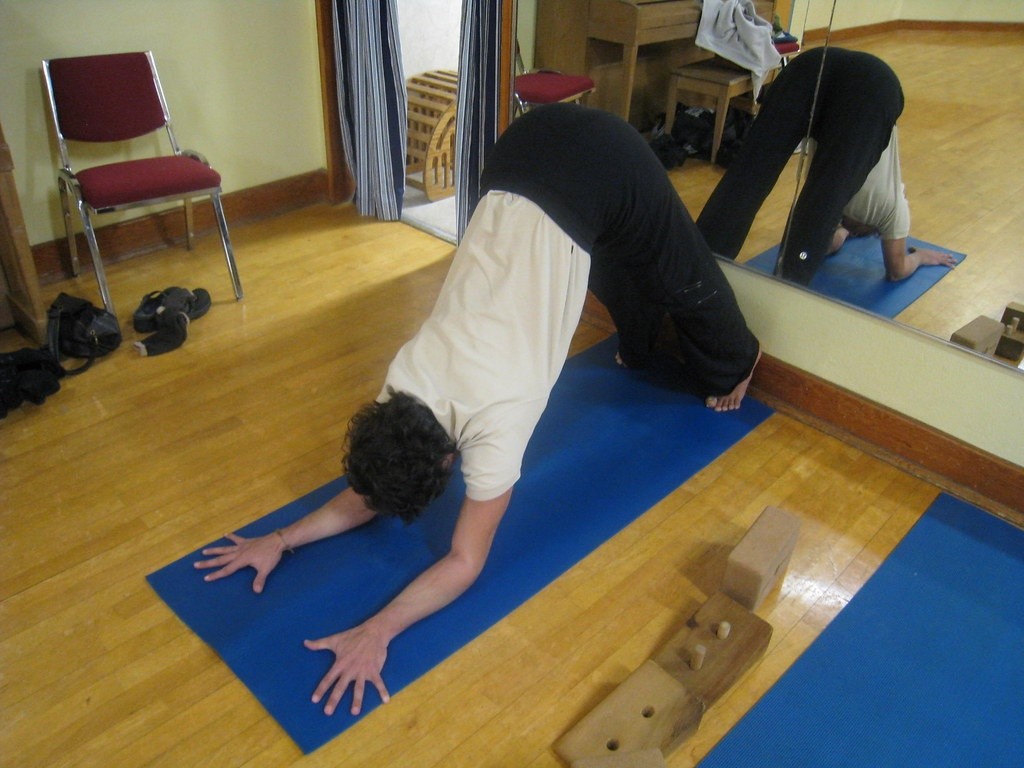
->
[696,46,957,285]
[193,103,762,715]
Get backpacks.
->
[47,293,124,375]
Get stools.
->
[663,59,779,166]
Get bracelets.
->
[277,529,295,554]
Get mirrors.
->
[507,0,1024,376]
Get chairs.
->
[42,48,244,317]
[512,38,598,120]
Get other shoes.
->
[153,287,213,331]
[132,290,166,334]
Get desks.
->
[587,0,777,134]
